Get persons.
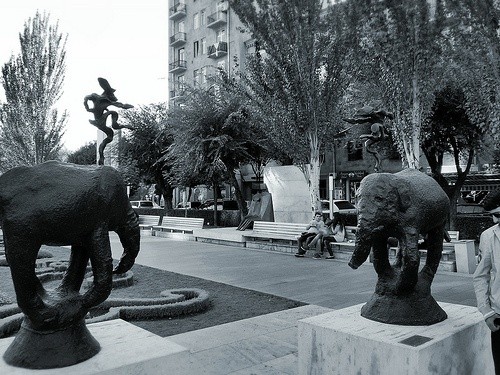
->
[295,211,325,257]
[312,212,346,260]
[301,212,340,260]
[472,206,500,375]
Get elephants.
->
[347,167,452,301]
[0,160,140,331]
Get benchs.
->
[243,220,311,246]
[137,215,161,234]
[328,226,459,260]
[151,215,205,234]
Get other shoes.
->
[325,256,335,260]
[295,252,305,257]
[301,245,310,251]
[312,255,321,260]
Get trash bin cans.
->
[450,238,477,274]
